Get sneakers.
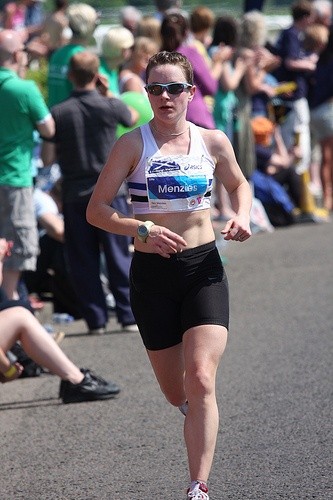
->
[186,481,209,500]
[59,368,121,404]
[178,400,188,416]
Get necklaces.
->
[150,123,189,135]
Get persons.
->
[156,14,237,222]
[6,0,333,308]
[0,238,121,404]
[0,29,56,301]
[40,52,140,334]
[86,51,252,500]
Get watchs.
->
[137,220,155,243]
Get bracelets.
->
[4,364,16,378]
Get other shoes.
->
[20,331,65,377]
[28,295,46,309]
[106,292,116,308]
[54,313,75,323]
[123,324,140,332]
[89,328,104,334]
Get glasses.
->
[144,82,193,97]
